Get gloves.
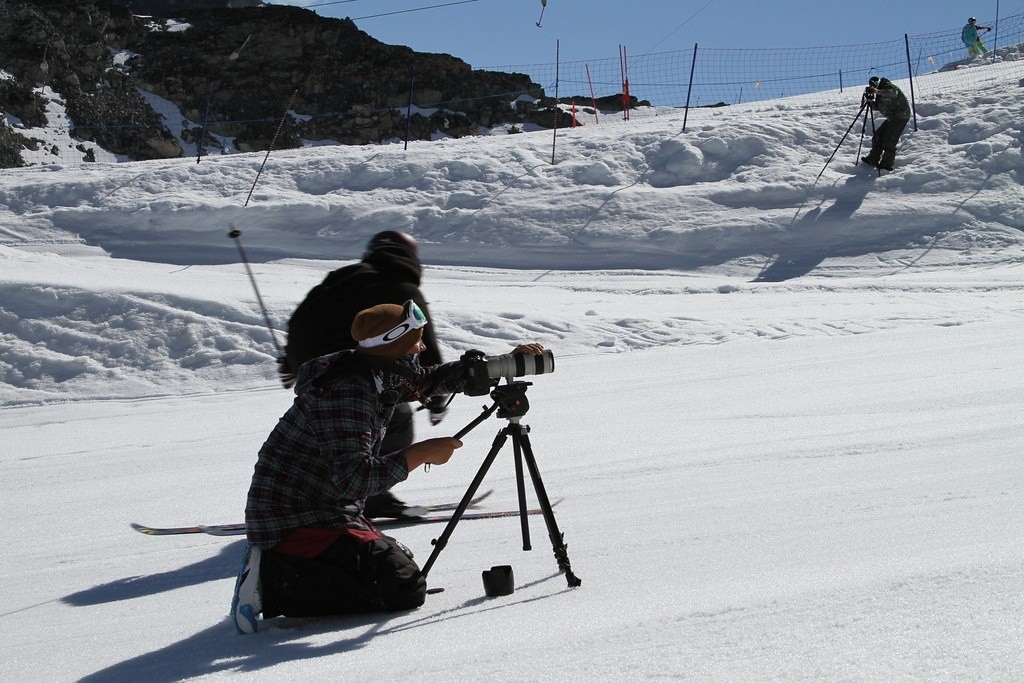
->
[987,26,992,32]
[278,373,296,389]
[864,85,878,101]
[430,410,446,426]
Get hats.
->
[869,76,879,88]
[350,303,424,358]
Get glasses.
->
[402,299,428,329]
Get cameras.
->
[865,87,874,99]
[460,349,555,397]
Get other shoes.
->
[876,164,895,170]
[861,157,878,166]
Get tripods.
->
[421,380,581,588]
[819,98,881,177]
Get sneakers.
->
[363,492,427,518]
[229,542,262,635]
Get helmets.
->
[362,230,421,278]
[968,17,976,22]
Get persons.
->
[276,230,449,518]
[230,301,544,635]
[861,76,911,170]
[961,17,991,56]
